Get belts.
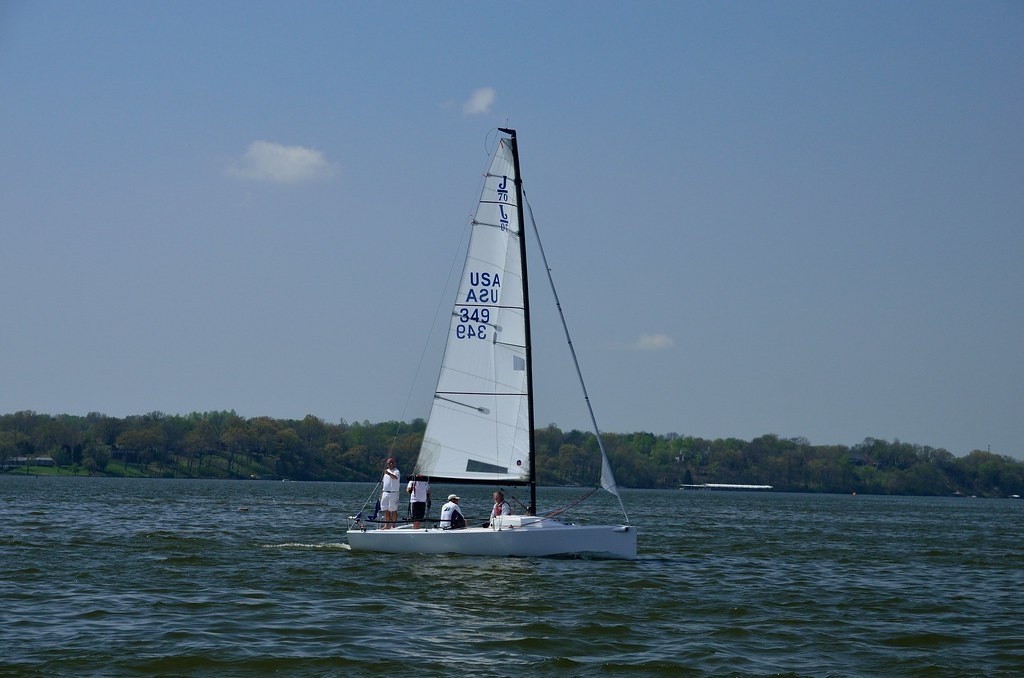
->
[383,491,396,493]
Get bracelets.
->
[388,473,390,475]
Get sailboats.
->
[346,126,638,561]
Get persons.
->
[406,468,431,529]
[489,490,512,528]
[379,457,401,530]
[439,493,467,530]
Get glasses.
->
[387,462,394,465]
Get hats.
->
[387,458,395,463]
[447,494,461,501]
